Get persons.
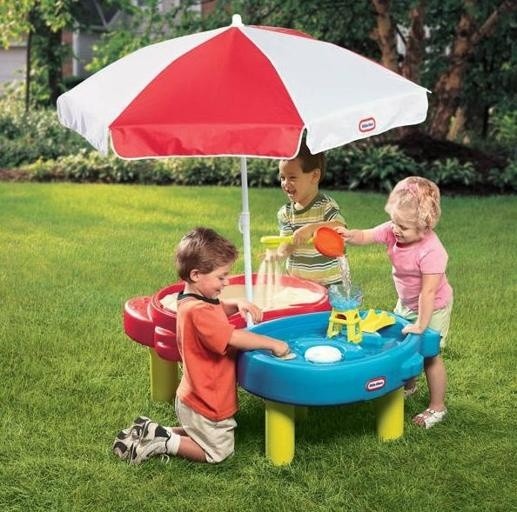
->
[257,138,350,289]
[113,228,291,466]
[334,176,456,431]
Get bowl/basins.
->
[315,226,346,257]
[326,286,362,309]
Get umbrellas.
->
[56,15,433,307]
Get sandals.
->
[413,407,447,429]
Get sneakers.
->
[113,416,171,465]
